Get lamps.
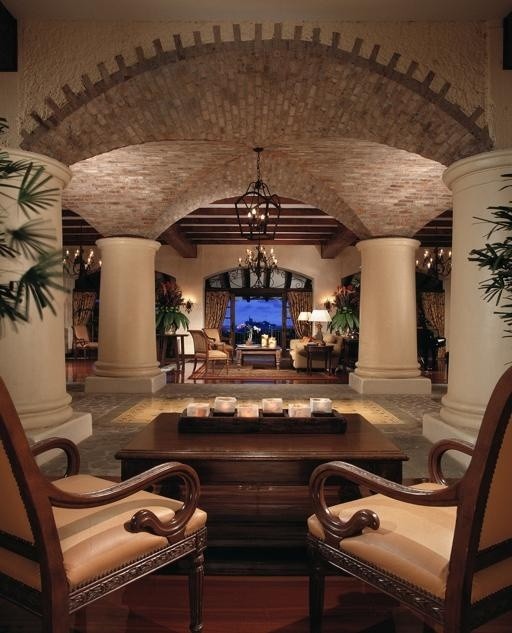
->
[182,296,195,314]
[415,221,452,280]
[233,245,280,282]
[63,216,102,278]
[234,144,282,242]
[296,311,311,336]
[320,296,336,312]
[308,309,333,345]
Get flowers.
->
[236,322,262,334]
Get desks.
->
[304,343,334,375]
[234,343,282,370]
[113,412,409,575]
[158,333,188,371]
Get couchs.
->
[289,333,345,371]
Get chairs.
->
[0,380,210,633]
[202,328,234,364]
[187,330,229,374]
[307,363,512,631]
[71,323,98,359]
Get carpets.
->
[188,359,337,379]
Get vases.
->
[245,330,253,345]
